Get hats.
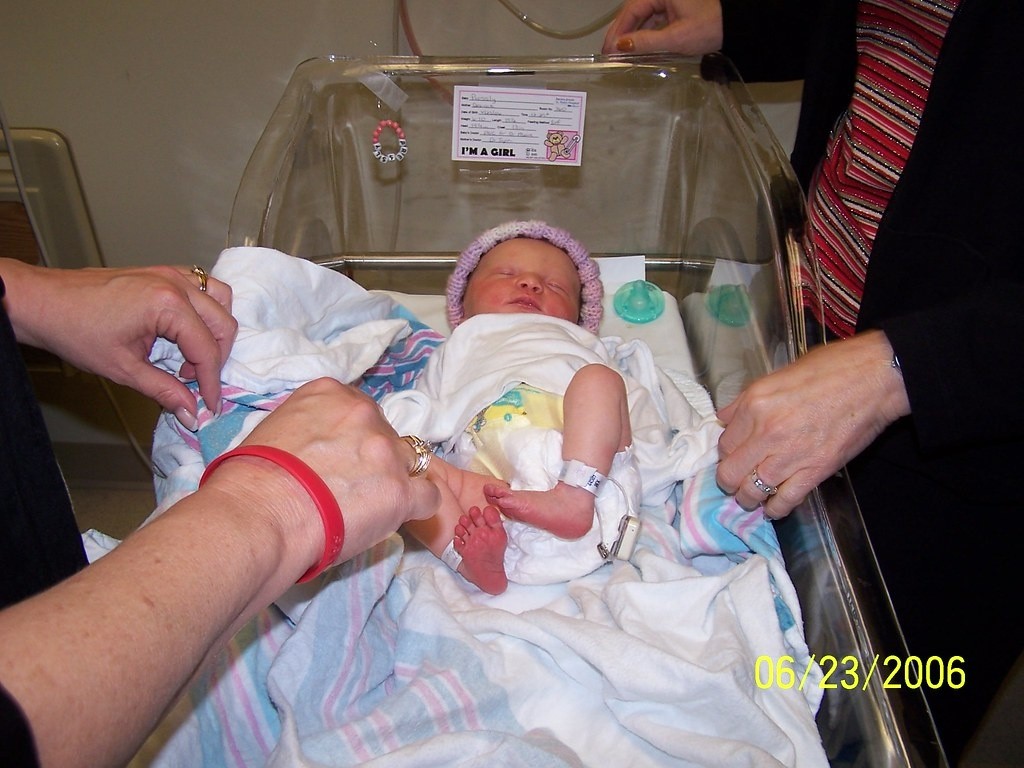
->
[445,220,605,333]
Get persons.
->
[379,222,638,595]
[604,2,1024,768]
[0,254,441,767]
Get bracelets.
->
[198,445,346,586]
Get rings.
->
[190,265,207,292]
[750,466,778,495]
[404,435,430,477]
